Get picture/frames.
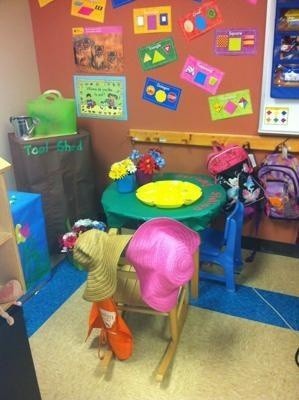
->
[73,75,127,121]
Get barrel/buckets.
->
[11,115,36,138]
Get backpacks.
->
[257,142,299,221]
[204,139,265,213]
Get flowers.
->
[128,146,167,174]
[108,159,137,180]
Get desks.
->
[100,173,226,231]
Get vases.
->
[136,170,152,185]
[117,175,136,193]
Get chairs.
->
[92,228,199,390]
[197,200,245,292]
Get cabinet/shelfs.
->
[0,158,27,312]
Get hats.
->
[125,217,202,311]
[73,228,134,302]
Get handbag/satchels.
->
[25,88,79,141]
